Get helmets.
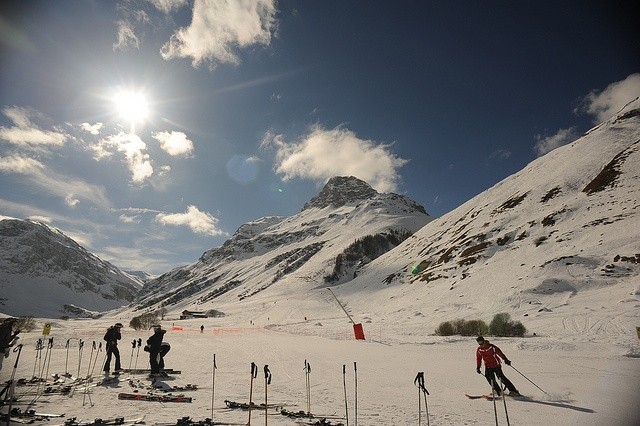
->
[3,317,16,325]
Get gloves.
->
[477,368,481,373]
[505,360,512,366]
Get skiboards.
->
[60,415,146,426]
[260,411,346,419]
[123,369,181,374]
[206,402,299,413]
[118,393,192,403]
[465,393,528,401]
[0,412,66,424]
[151,421,249,426]
[0,372,124,406]
[125,377,152,392]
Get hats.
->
[152,324,161,331]
[476,336,484,342]
[116,323,122,327]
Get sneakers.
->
[509,390,520,395]
[490,389,503,396]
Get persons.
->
[476,335,518,396]
[148,324,166,376]
[144,342,171,372]
[103,323,124,372]
